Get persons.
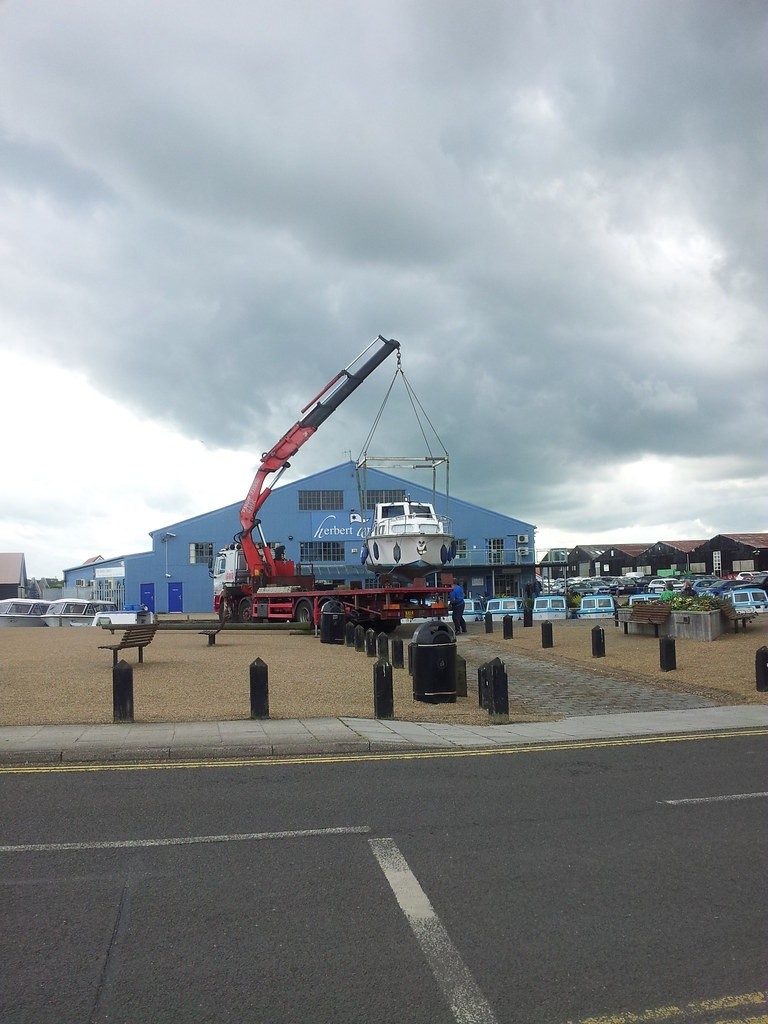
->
[447,577,468,636]
[532,579,543,598]
[680,581,698,598]
[524,580,534,600]
[659,582,678,603]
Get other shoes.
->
[455,630,460,635]
[461,629,468,632]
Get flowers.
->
[565,589,581,609]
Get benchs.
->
[197,617,227,645]
[97,622,160,668]
[716,600,756,634]
[614,603,673,638]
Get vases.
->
[569,608,580,619]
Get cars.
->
[539,568,768,598]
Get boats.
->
[724,586,768,615]
[37,597,118,628]
[532,595,573,620]
[481,596,526,622]
[441,597,484,622]
[362,492,458,578]
[399,598,439,624]
[629,594,662,607]
[69,603,155,628]
[0,596,53,628]
[576,593,616,619]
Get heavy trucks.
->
[208,539,457,638]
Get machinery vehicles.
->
[229,334,450,592]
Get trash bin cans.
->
[409,621,458,703]
[320,601,346,645]
